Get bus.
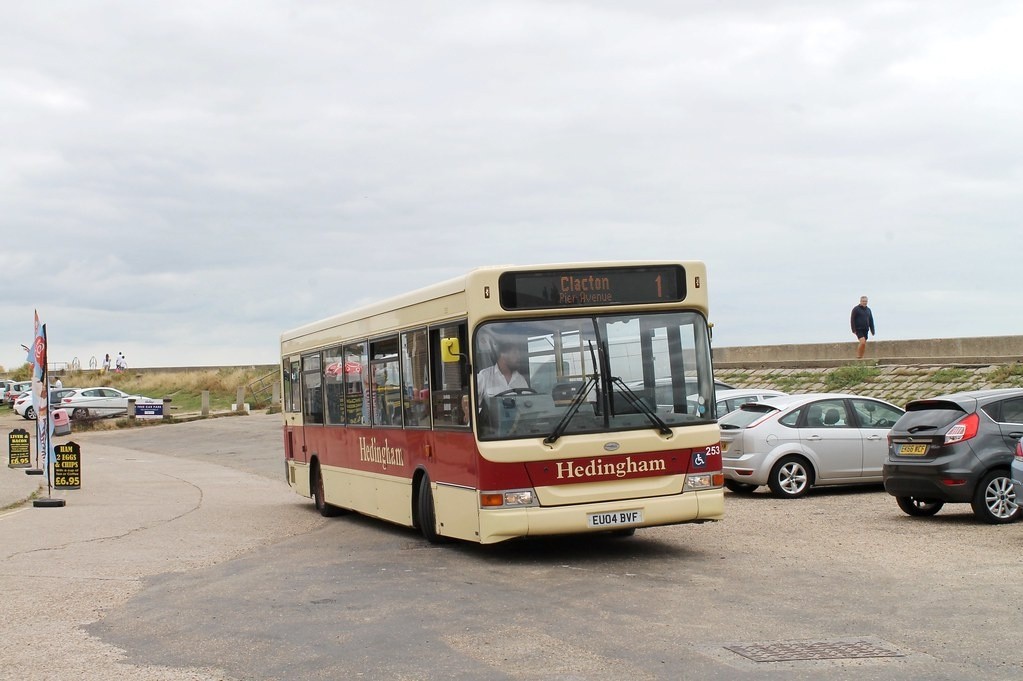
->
[278,260,726,547]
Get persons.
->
[103,354,110,371]
[851,296,875,358]
[54,377,62,388]
[119,356,127,371]
[467,336,531,427]
[116,352,122,370]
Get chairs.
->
[809,406,824,426]
[824,409,840,426]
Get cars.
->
[60,386,151,421]
[670,389,788,420]
[717,392,906,499]
[616,378,735,413]
[882,388,1023,524]
[0,378,83,420]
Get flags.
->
[26,310,58,487]
